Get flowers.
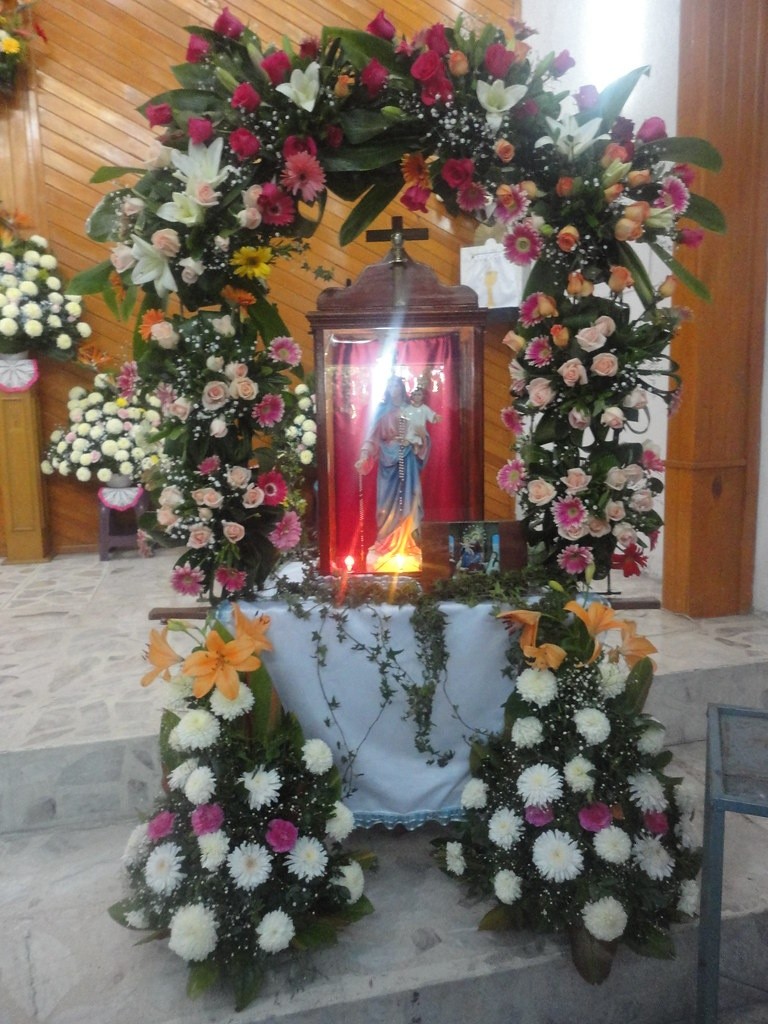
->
[0,7,707,985]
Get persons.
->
[355,376,429,572]
[400,385,443,459]
[477,252,509,306]
